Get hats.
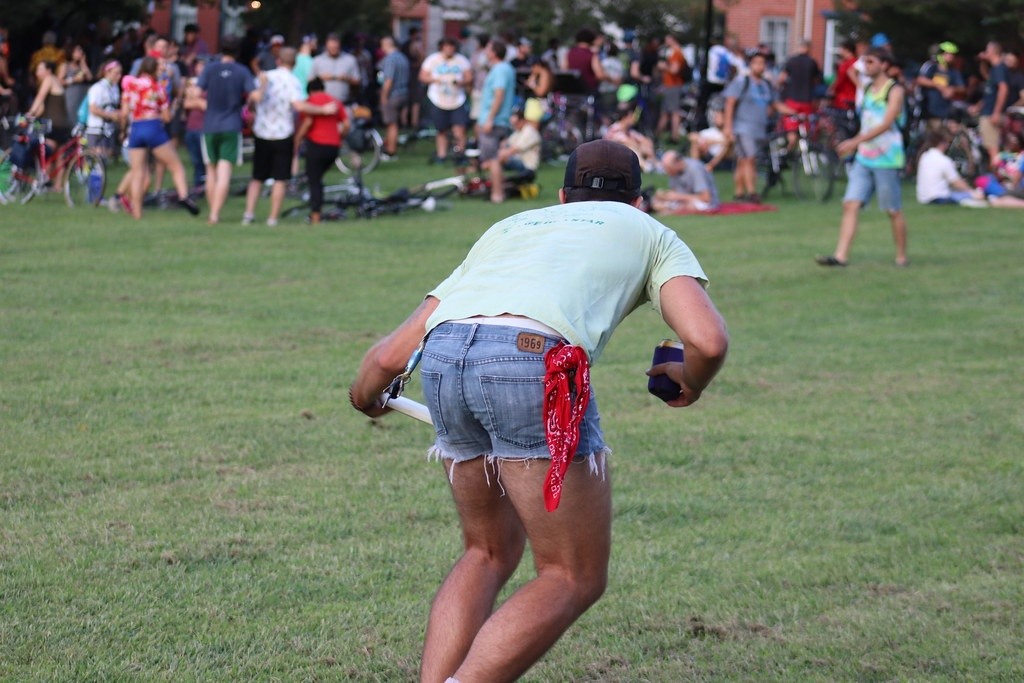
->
[870,34,888,49]
[183,23,200,32]
[744,47,766,65]
[270,35,285,46]
[564,139,642,192]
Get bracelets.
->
[348,384,376,412]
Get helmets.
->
[939,42,958,54]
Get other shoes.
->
[815,256,849,267]
[178,197,200,215]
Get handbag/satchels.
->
[10,132,33,168]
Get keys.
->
[380,378,405,408]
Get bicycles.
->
[755,112,835,204]
[0,118,105,209]
[298,120,460,219]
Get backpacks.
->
[678,60,694,84]
[866,82,912,149]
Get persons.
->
[916,39,1024,208]
[813,47,910,269]
[417,30,905,213]
[0,22,429,228]
[349,139,728,683]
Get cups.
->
[646,339,686,399]
